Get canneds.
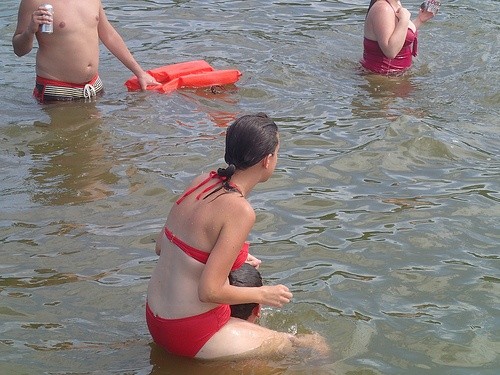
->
[40,4,54,34]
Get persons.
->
[145,111,325,364]
[359,0,441,76]
[228,262,265,322]
[14,1,160,104]
